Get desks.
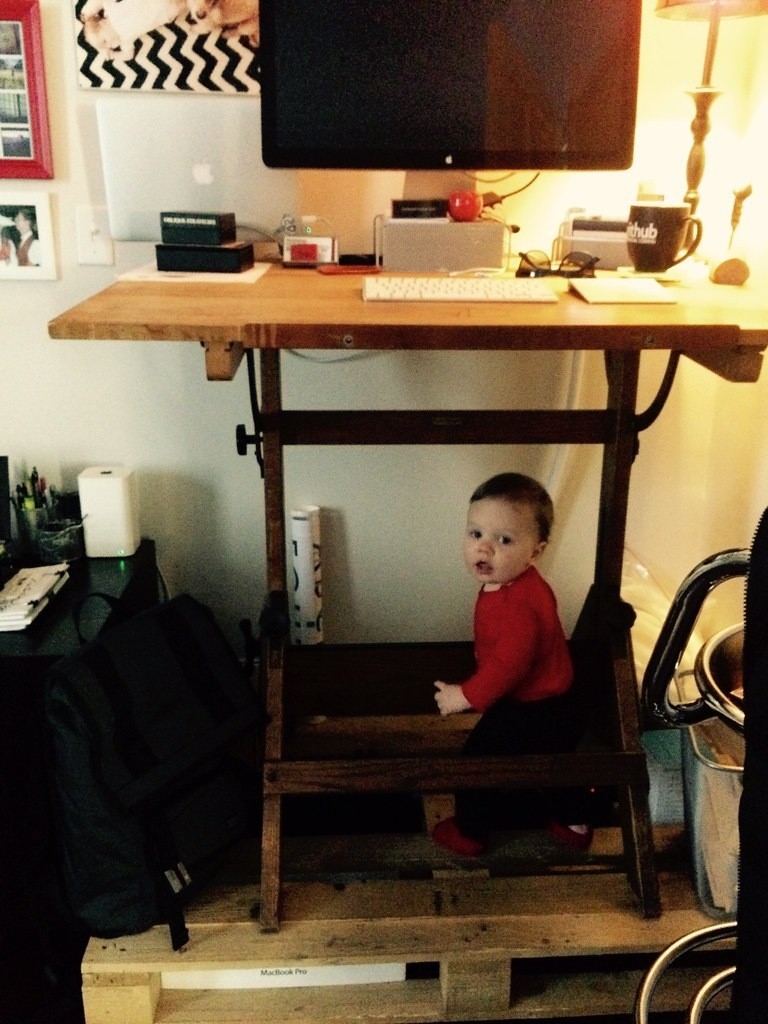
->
[48,263,768,932]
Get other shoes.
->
[432,816,482,853]
[554,825,592,851]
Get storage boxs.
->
[156,243,254,274]
[160,211,237,245]
[674,668,746,920]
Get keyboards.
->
[362,276,559,302]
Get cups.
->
[627,204,702,273]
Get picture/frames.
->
[0,0,54,179]
[0,190,58,281]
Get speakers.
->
[77,465,141,557]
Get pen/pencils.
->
[11,464,55,510]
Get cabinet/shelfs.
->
[0,538,156,962]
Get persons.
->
[79,0,199,66]
[0,209,42,267]
[428,471,595,866]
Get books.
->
[0,564,71,631]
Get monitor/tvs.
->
[258,0,643,204]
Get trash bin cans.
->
[673,668,747,921]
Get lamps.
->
[653,0,768,251]
